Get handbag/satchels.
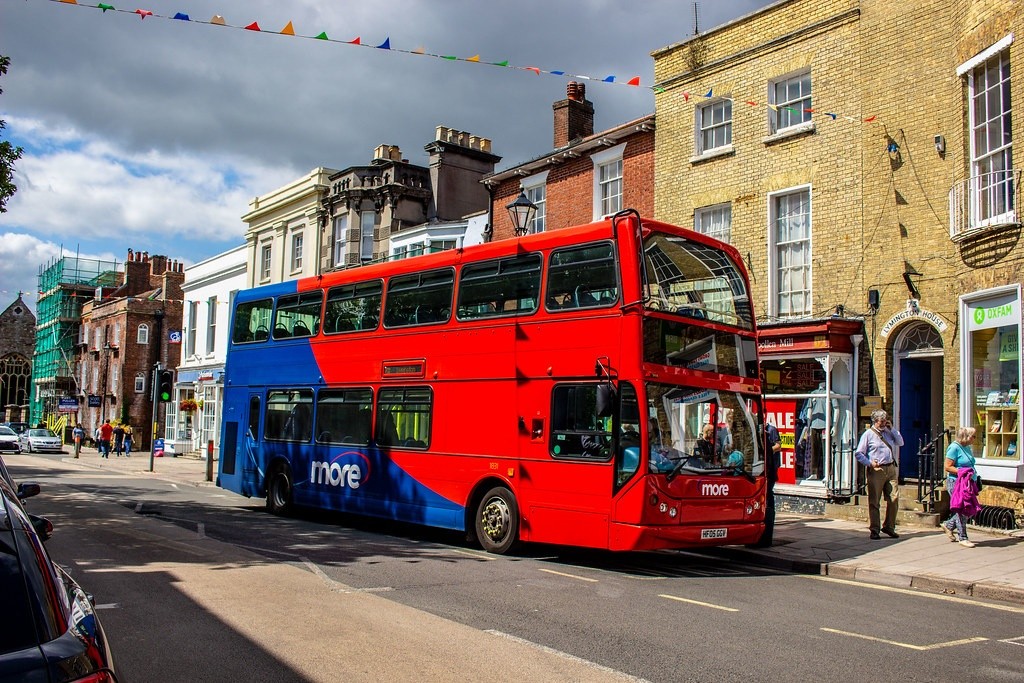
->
[977,476,982,492]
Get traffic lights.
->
[158,369,175,404]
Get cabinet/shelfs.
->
[985,406,1021,459]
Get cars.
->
[0,426,23,455]
[4,422,30,435]
[0,457,115,682]
[18,428,63,453]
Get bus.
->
[214,209,768,554]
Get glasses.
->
[972,435,976,440]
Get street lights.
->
[99,340,120,427]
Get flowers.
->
[181,397,199,413]
[88,347,96,351]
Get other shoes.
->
[744,543,772,549]
[806,475,817,480]
[941,523,956,541]
[822,477,830,483]
[959,540,975,547]
[881,527,899,538]
[870,530,881,539]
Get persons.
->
[122,429,135,457]
[855,409,903,539]
[941,428,978,547]
[624,424,636,436]
[96,420,113,458]
[382,412,400,445]
[680,292,704,317]
[724,446,745,475]
[581,433,607,458]
[111,424,128,457]
[691,413,735,463]
[746,423,781,549]
[649,418,664,444]
[797,382,840,479]
[37,420,44,429]
[73,423,83,453]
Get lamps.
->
[778,359,786,365]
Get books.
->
[987,389,1019,406]
[1007,440,1016,456]
[990,420,1002,433]
[1011,419,1017,432]
[994,443,1001,456]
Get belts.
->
[878,462,894,467]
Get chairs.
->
[547,284,703,317]
[241,306,450,341]
[296,428,426,447]
